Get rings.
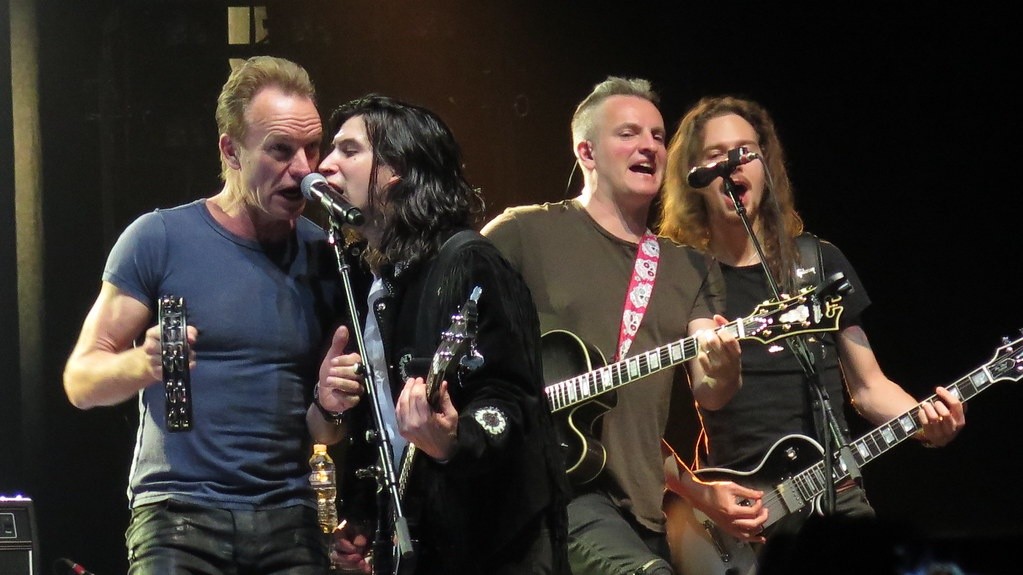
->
[705,348,713,356]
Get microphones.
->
[687,153,759,189]
[301,173,365,227]
[63,558,94,575]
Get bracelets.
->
[313,383,344,427]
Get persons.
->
[657,98,966,575]
[480,75,742,575]
[317,93,569,574]
[63,55,366,575]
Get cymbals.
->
[156,291,195,434]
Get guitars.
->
[363,284,488,574]
[661,326,1023,574]
[534,267,858,489]
